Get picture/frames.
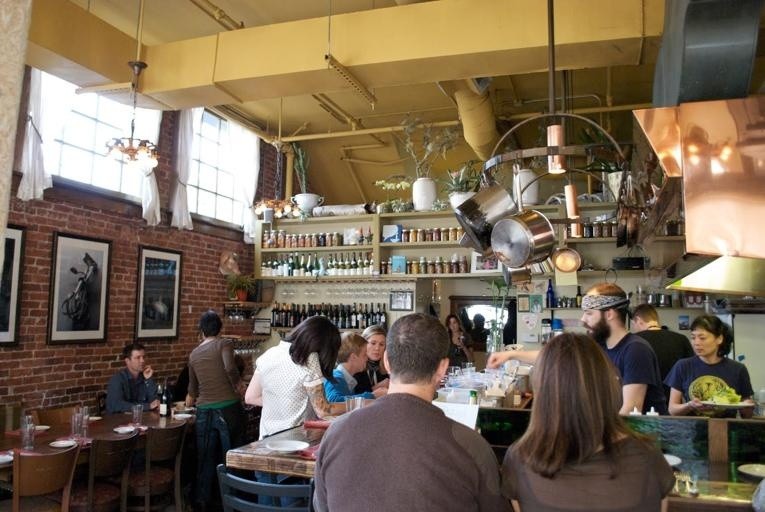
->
[1,223,183,349]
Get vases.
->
[291,193,324,217]
[449,191,476,210]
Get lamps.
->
[254,97,302,215]
[98,1,162,170]
[547,0,581,237]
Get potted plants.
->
[390,116,461,211]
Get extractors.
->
[659,253,764,296]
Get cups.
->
[20,415,35,450]
[71,406,90,441]
[131,405,143,423]
[345,397,365,413]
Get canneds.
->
[400,226,466,243]
[425,259,435,275]
[410,260,420,274]
[284,231,342,248]
[380,259,387,274]
[405,261,411,273]
[441,261,450,274]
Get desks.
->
[662,478,765,512]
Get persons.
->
[312,313,511,512]
[447,315,473,366]
[107,344,161,412]
[632,303,759,417]
[326,332,385,401]
[244,316,374,504]
[470,315,490,352]
[580,284,668,414]
[356,326,387,393]
[500,333,676,512]
[175,310,243,509]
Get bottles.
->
[577,286,581,306]
[547,279,554,308]
[271,301,388,329]
[261,252,373,277]
[160,379,171,417]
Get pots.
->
[456,180,519,250]
[502,262,532,290]
[552,225,582,273]
[491,163,555,268]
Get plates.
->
[174,414,191,420]
[663,453,682,466]
[266,441,310,455]
[48,440,76,448]
[171,408,192,413]
[90,416,103,421]
[0,455,14,464]
[36,425,51,431]
[113,427,134,433]
[700,401,754,409]
[736,463,764,478]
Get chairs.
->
[1,397,197,512]
[217,464,315,512]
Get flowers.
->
[437,162,478,191]
[372,174,409,213]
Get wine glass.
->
[239,308,246,320]
[228,309,233,320]
[233,308,240,320]
[234,341,265,355]
